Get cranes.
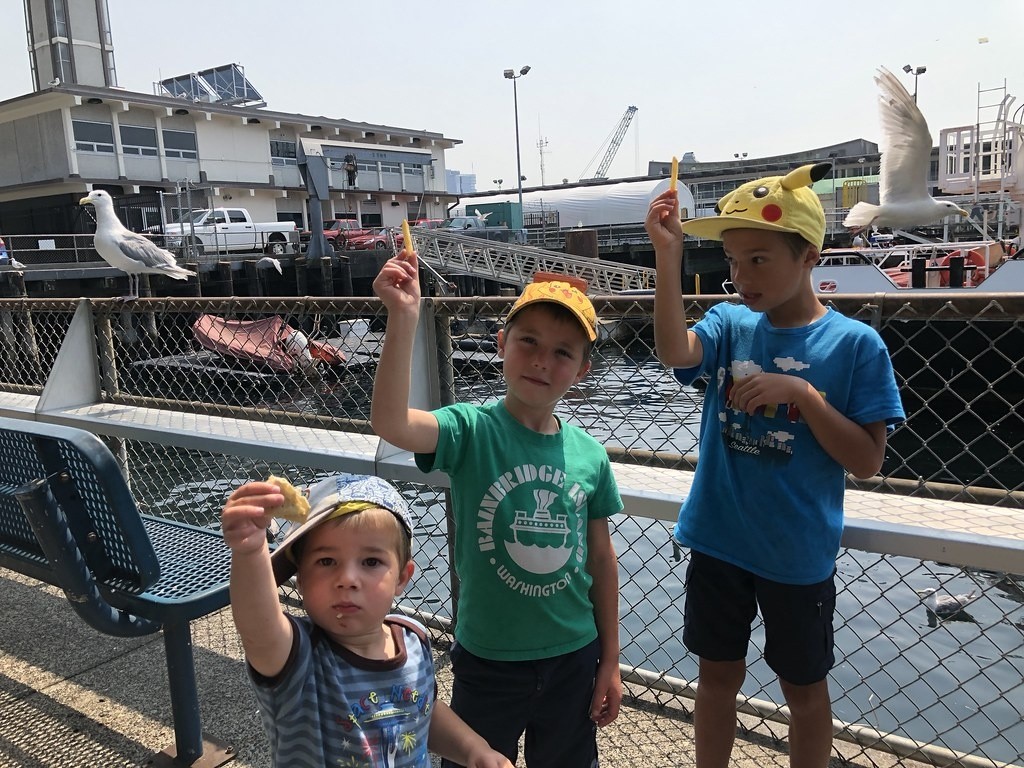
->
[575,104,639,183]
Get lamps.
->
[311,126,321,130]
[175,109,189,115]
[365,132,375,137]
[413,138,420,142]
[87,98,103,104]
[248,118,260,124]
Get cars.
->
[346,226,409,251]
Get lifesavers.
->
[335,234,346,249]
[940,250,985,287]
[1005,245,1016,256]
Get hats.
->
[268,474,412,589]
[680,162,833,252]
[504,280,599,342]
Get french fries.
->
[670,157,678,193]
[401,219,413,256]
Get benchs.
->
[0,417,298,767]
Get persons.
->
[220,473,515,768]
[370,249,625,768]
[646,161,906,768]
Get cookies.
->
[265,474,310,524]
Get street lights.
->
[903,64,926,106]
[503,65,531,228]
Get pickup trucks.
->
[139,207,299,257]
[433,217,510,243]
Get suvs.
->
[400,219,443,237]
[299,217,362,250]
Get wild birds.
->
[917,586,979,612]
[283,331,323,381]
[79,189,198,304]
[474,209,493,221]
[841,65,971,249]
[577,220,583,228]
[256,256,283,275]
[8,257,27,271]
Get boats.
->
[192,312,347,375]
